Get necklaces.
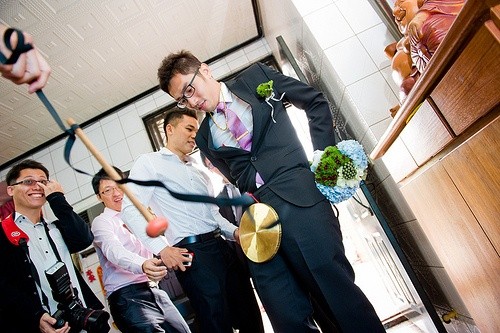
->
[208,82,228,131]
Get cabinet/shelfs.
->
[370,0,500,333]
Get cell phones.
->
[181,253,194,268]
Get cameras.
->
[44,261,111,333]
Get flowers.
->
[309,138,371,205]
[256,80,273,97]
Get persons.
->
[0,159,105,333]
[92,165,193,333]
[120,108,266,333]
[158,52,388,333]
